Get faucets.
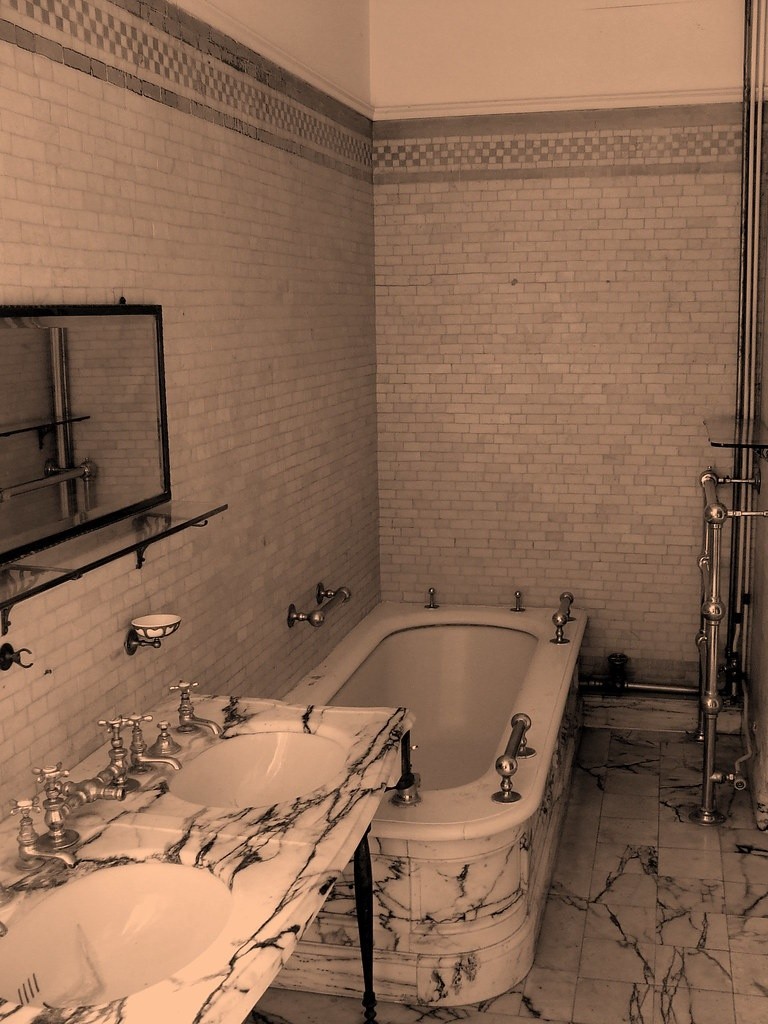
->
[8,796,76,871]
[168,677,224,735]
[120,712,183,775]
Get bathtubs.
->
[269,599,588,1006]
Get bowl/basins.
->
[131,614,182,638]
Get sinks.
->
[164,720,351,809]
[0,854,233,1009]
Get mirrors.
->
[0,305,171,567]
